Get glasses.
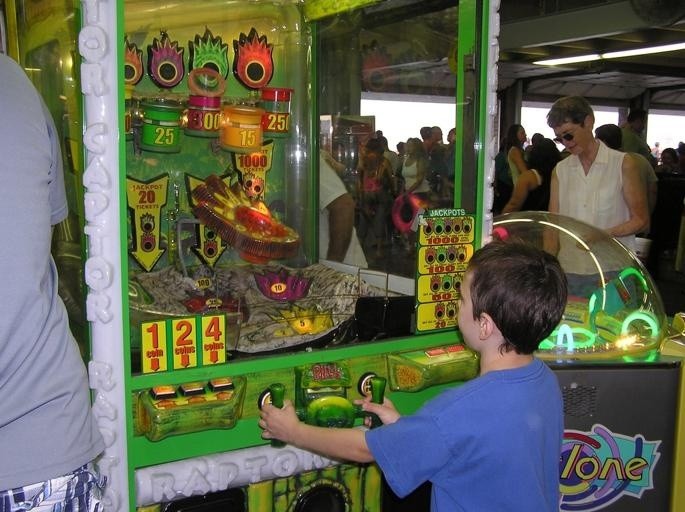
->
[553,123,583,145]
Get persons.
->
[258,232,570,512]
[0,45,111,511]
